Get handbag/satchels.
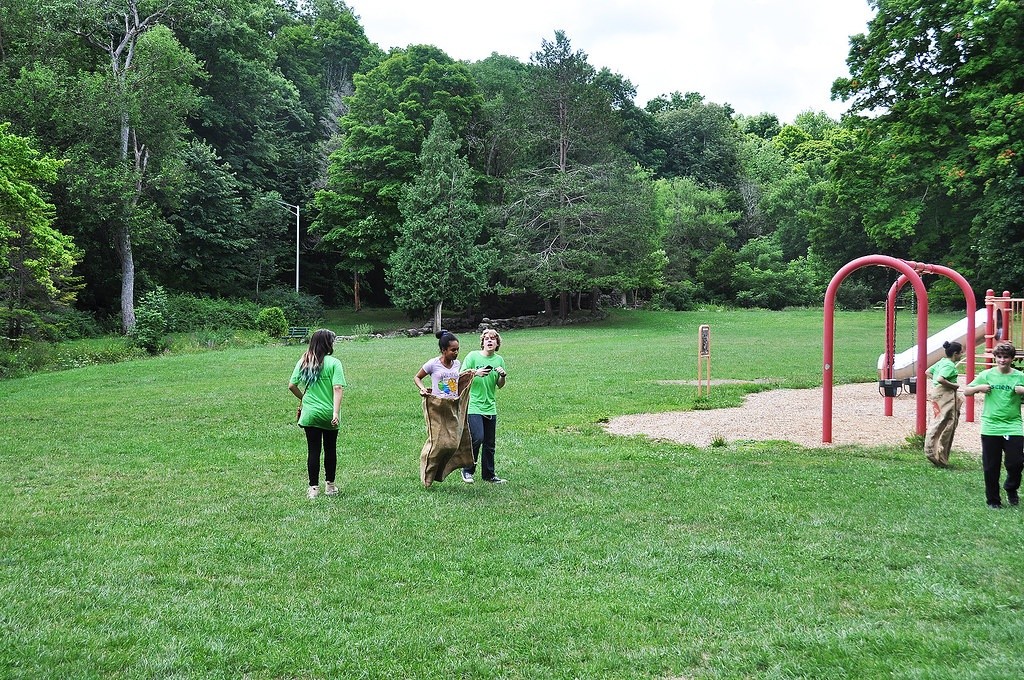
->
[297,408,303,428]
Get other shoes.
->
[1005,490,1020,506]
[984,501,1005,512]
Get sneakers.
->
[461,470,475,484]
[306,485,320,500]
[488,476,507,484]
[324,485,338,495]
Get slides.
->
[877,307,998,381]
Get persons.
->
[923,341,964,466]
[414,330,475,486]
[289,329,347,499]
[964,343,1024,509]
[461,329,507,484]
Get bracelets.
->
[500,374,506,377]
